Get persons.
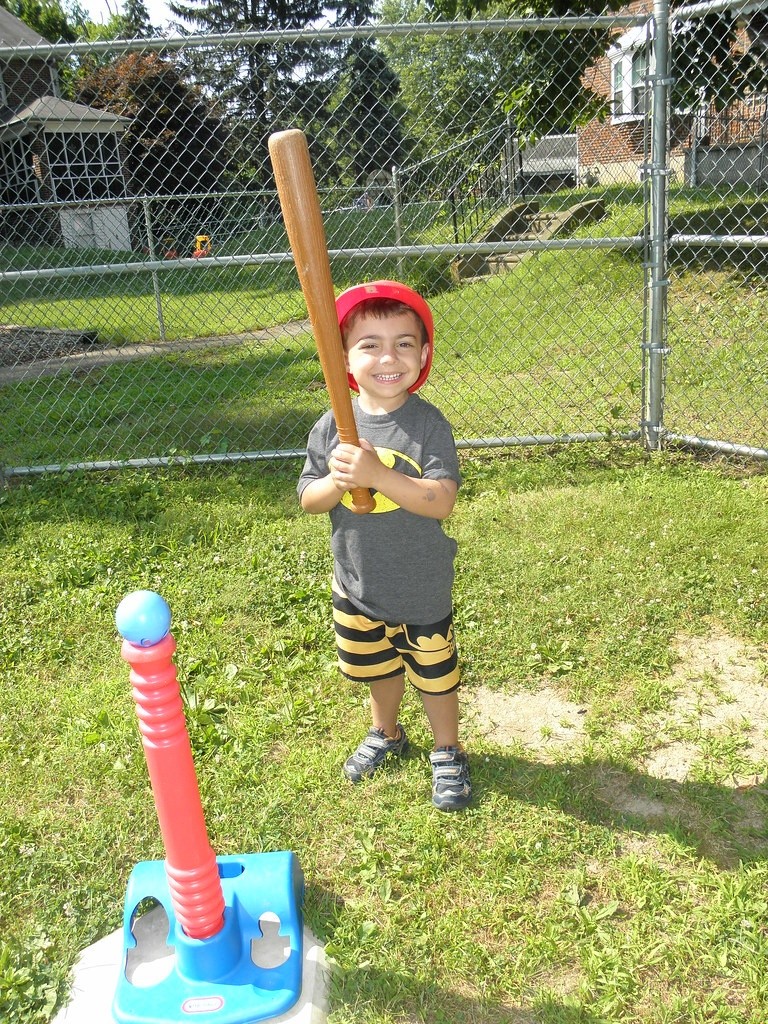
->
[297,278,471,811]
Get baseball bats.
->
[262,121,380,520]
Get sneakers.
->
[429,743,473,811]
[343,723,408,783]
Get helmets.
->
[334,280,433,392]
